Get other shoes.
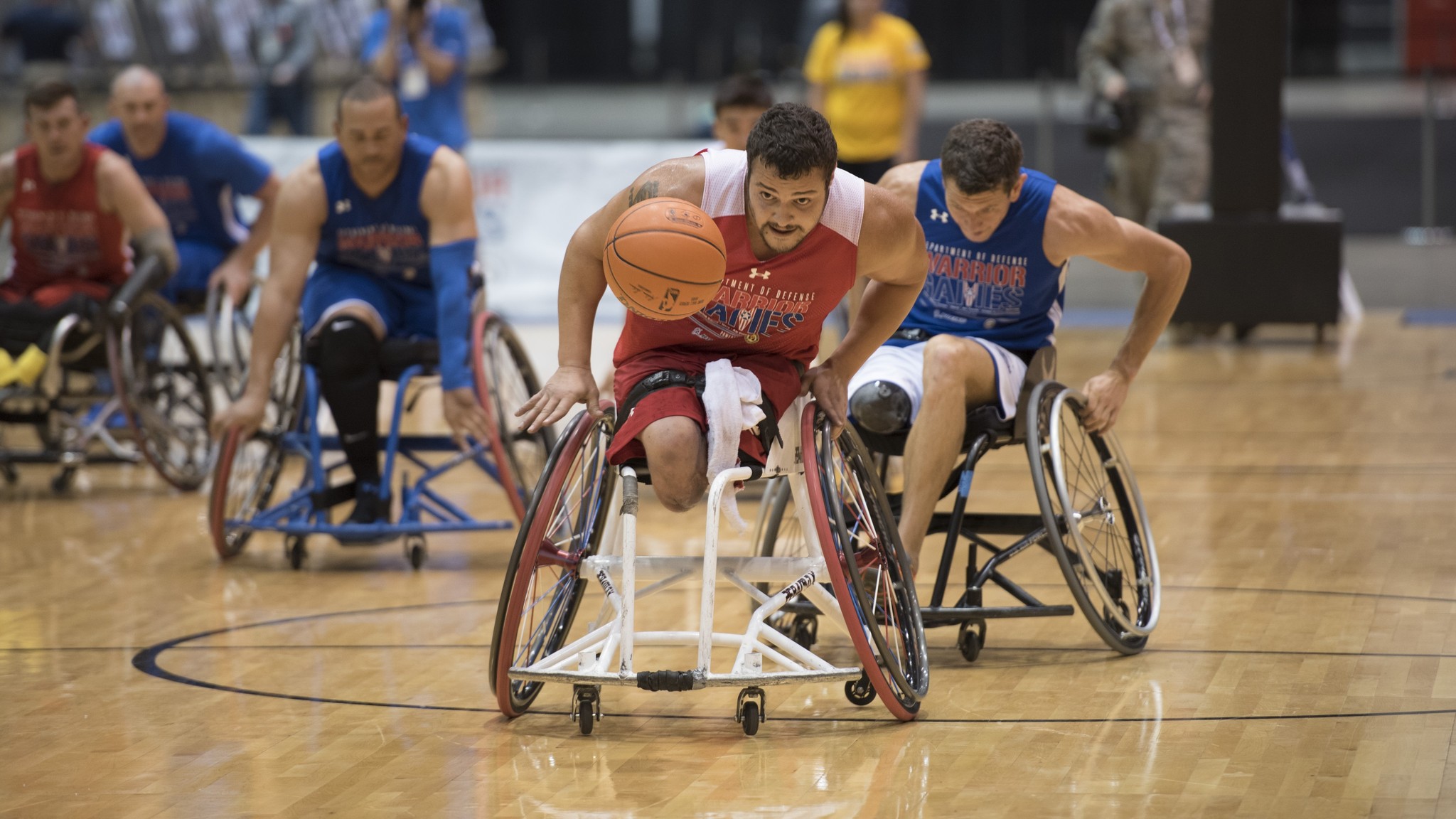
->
[333,483,393,524]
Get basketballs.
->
[603,197,728,322]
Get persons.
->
[845,115,1192,593]
[714,0,931,185]
[86,62,286,465]
[350,0,502,171]
[511,101,925,511]
[240,0,329,138]
[213,72,493,548]
[0,80,173,404]
[1080,0,1218,239]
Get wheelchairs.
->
[747,342,1161,656]
[0,241,298,497]
[208,269,575,570]
[488,373,934,737]
